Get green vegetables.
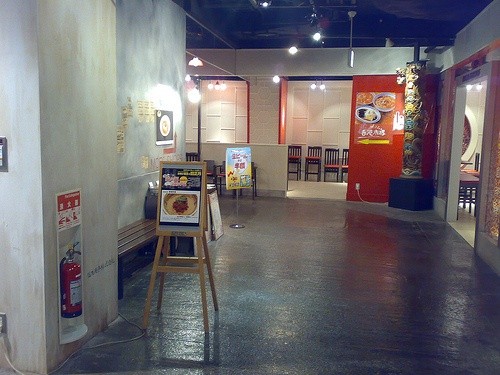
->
[175,195,186,202]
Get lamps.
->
[385,38,393,48]
[188,57,204,67]
[346,11,356,68]
[311,80,325,90]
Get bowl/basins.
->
[372,93,396,113]
[162,194,199,216]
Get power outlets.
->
[0,313,7,333]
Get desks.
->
[458,161,480,216]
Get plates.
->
[355,105,381,123]
[160,115,171,137]
[356,92,375,105]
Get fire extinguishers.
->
[59,241,83,318]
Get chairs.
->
[341,149,349,183]
[464,152,479,173]
[185,151,257,200]
[324,148,340,182]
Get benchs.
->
[118,218,176,299]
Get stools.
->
[305,146,322,182]
[286,145,301,181]
